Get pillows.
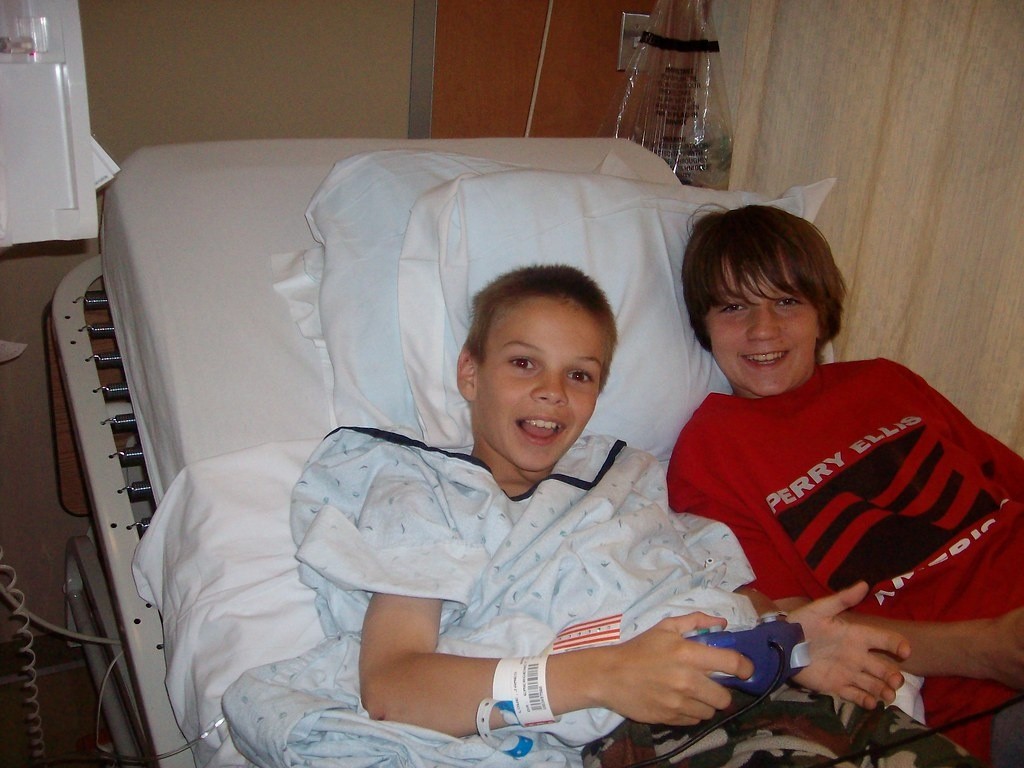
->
[271,151,837,445]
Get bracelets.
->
[477,653,561,757]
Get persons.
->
[663,206,1024,768]
[357,263,982,768]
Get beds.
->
[48,138,685,768]
